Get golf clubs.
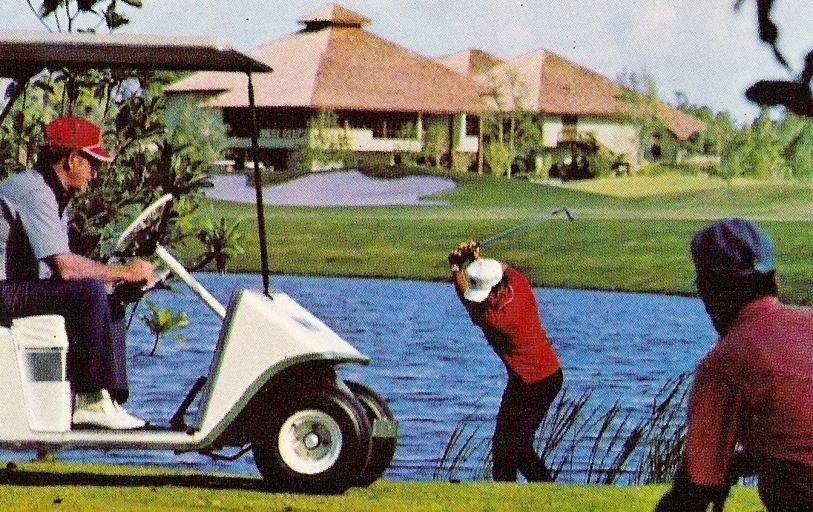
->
[478,206,574,245]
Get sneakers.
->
[73,389,145,430]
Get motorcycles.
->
[0,14,393,496]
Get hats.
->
[691,218,775,280]
[463,259,503,303]
[44,116,113,162]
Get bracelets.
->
[451,265,460,273]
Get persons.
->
[0,117,153,434]
[654,216,811,510]
[447,239,561,482]
